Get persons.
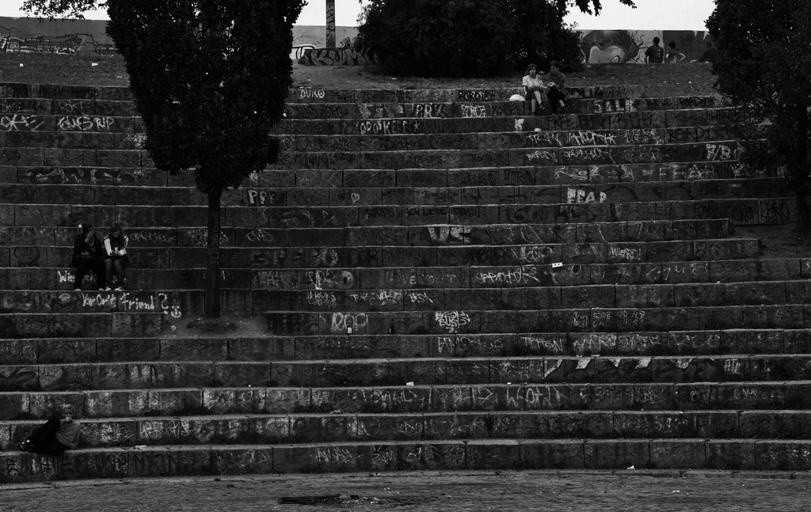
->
[19,403,83,458]
[546,60,568,116]
[72,222,107,292]
[100,222,131,292]
[664,39,687,64]
[644,36,667,65]
[689,40,716,65]
[520,62,548,116]
